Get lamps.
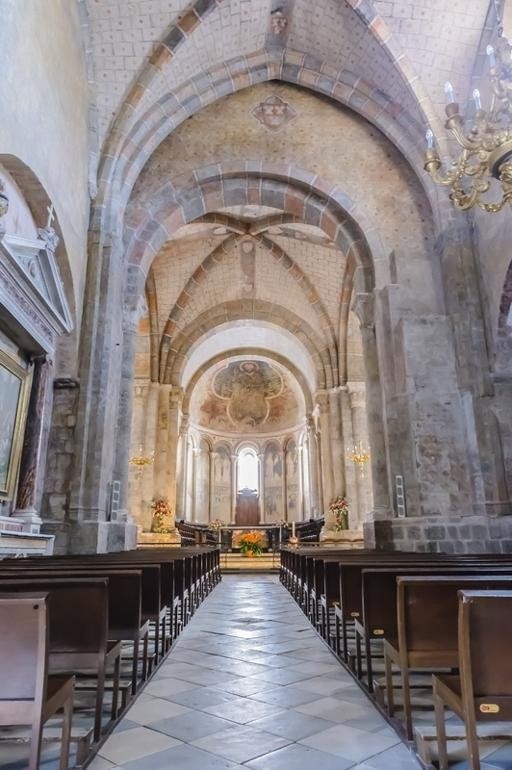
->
[421,1,512,214]
[346,446,368,472]
[129,445,157,468]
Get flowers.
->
[237,533,263,555]
[151,494,174,531]
[328,496,350,530]
